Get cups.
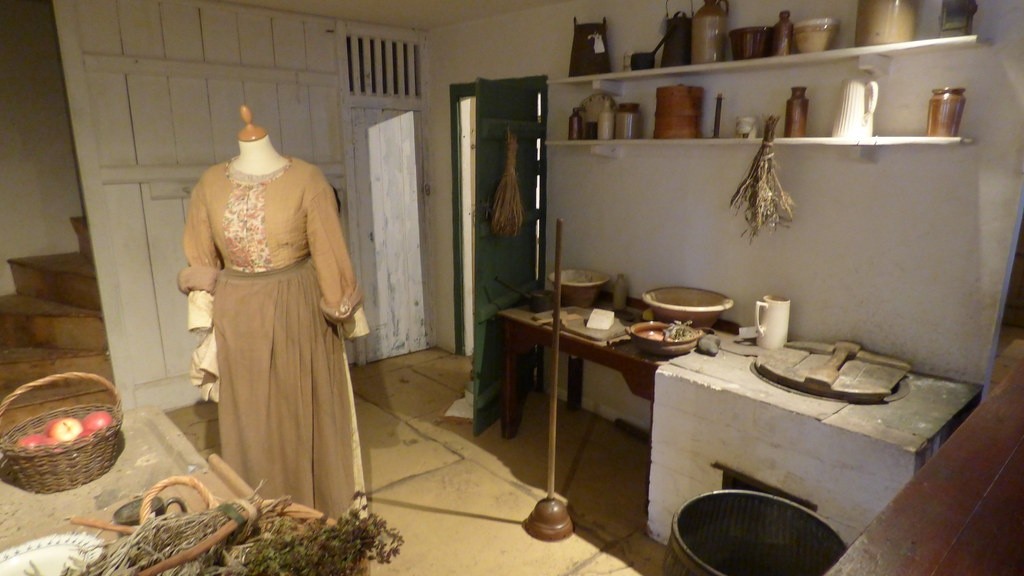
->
[114,495,187,537]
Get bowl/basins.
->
[642,288,734,330]
[550,271,609,308]
[729,27,773,59]
[793,18,839,51]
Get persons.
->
[176,104,370,518]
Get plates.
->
[0,533,106,576]
[579,93,616,122]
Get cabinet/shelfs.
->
[539,31,992,148]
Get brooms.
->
[487,127,528,239]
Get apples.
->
[14,410,109,448]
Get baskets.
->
[141,475,371,576]
[0,372,123,494]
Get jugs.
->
[569,0,977,77]
[754,294,790,349]
[832,81,880,139]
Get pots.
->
[756,342,909,404]
[625,321,715,356]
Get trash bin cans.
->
[663,489,847,576]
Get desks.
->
[0,407,244,576]
[497,295,743,439]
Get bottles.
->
[568,85,966,140]
[612,275,626,309]
